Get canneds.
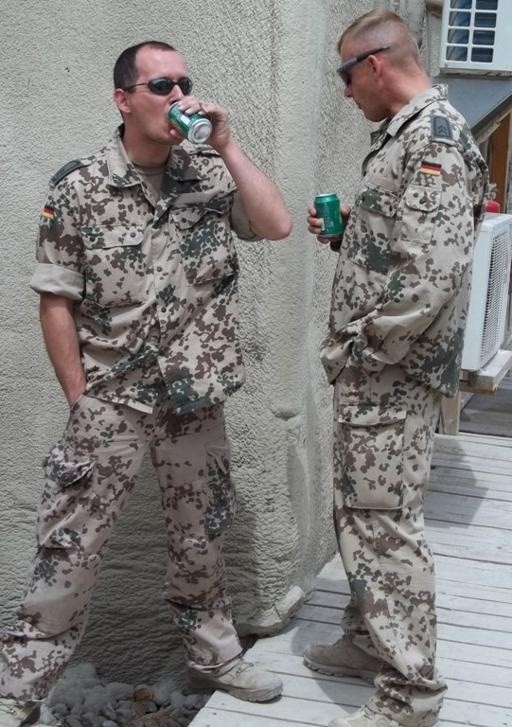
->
[167,101,213,144]
[314,192,345,238]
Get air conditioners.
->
[460,210,511,374]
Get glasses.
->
[123,77,194,96]
[336,45,390,85]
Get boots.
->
[188,657,283,703]
[304,633,389,684]
[0,697,41,727]
[328,694,441,727]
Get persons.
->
[0,41,293,727]
[307,9,492,727]
